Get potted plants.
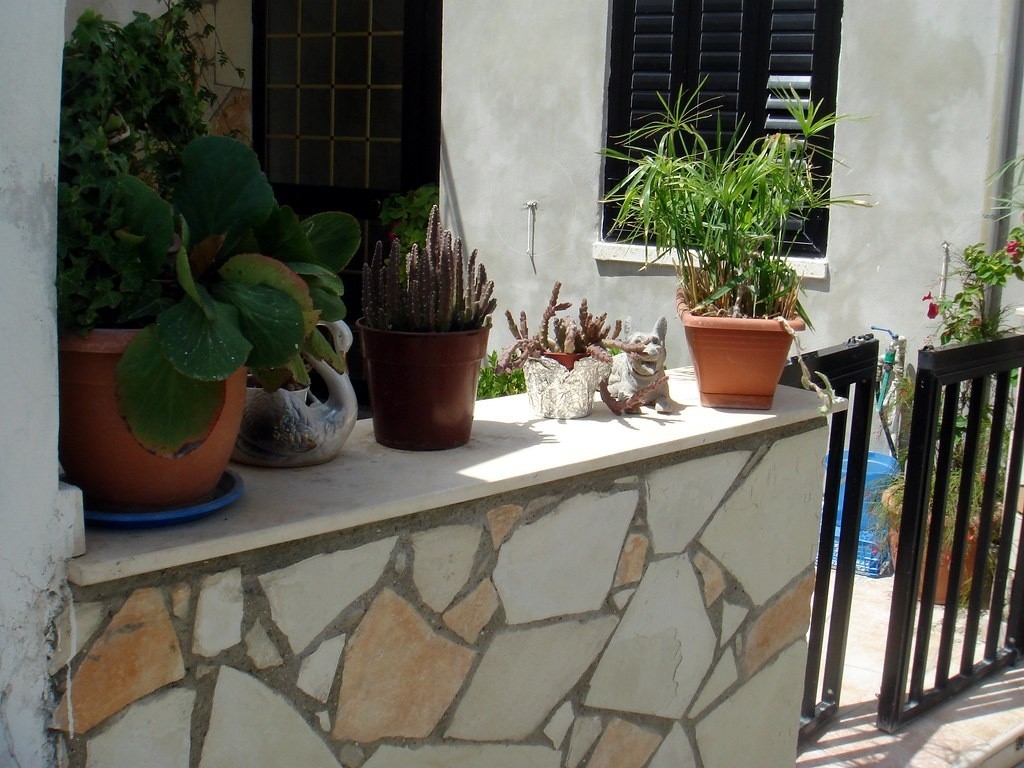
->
[864,244,1012,605]
[357,203,498,452]
[594,73,878,410]
[494,280,668,419]
[57,135,366,512]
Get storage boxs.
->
[816,451,903,576]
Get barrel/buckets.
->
[824,450,902,532]
